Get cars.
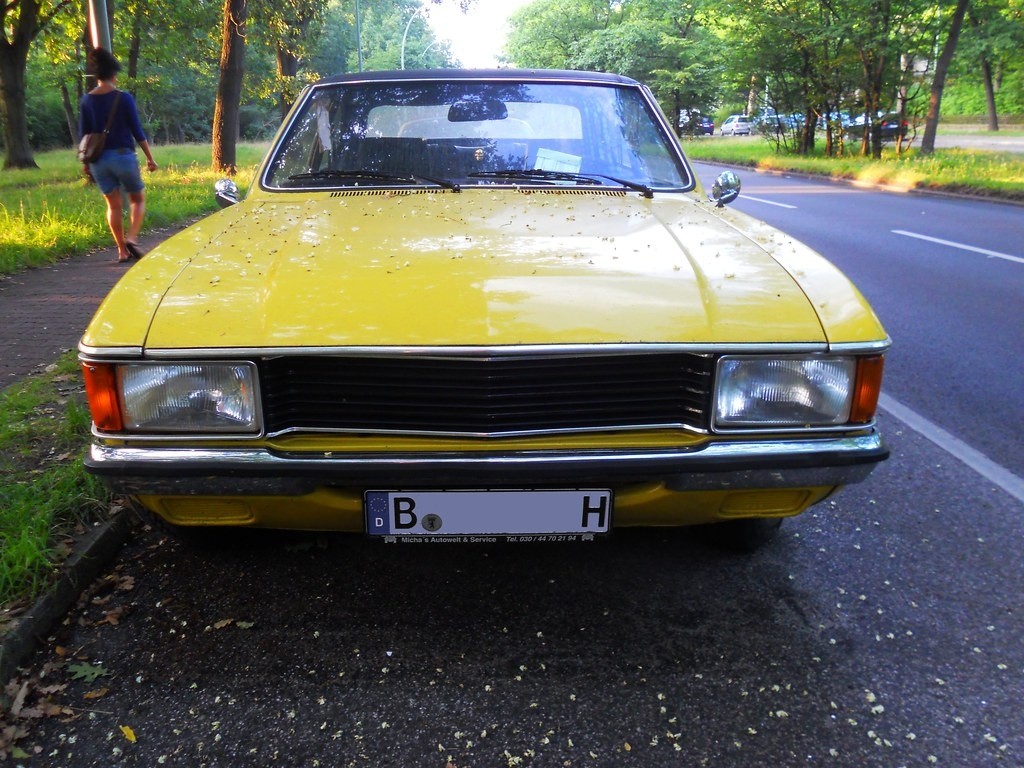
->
[844,111,908,141]
[684,117,715,136]
[721,115,755,136]
[753,106,853,133]
[77,69,892,544]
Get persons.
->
[77,47,159,262]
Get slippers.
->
[118,248,131,263]
[126,240,145,259]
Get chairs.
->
[337,137,429,177]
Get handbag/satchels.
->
[76,130,107,163]
